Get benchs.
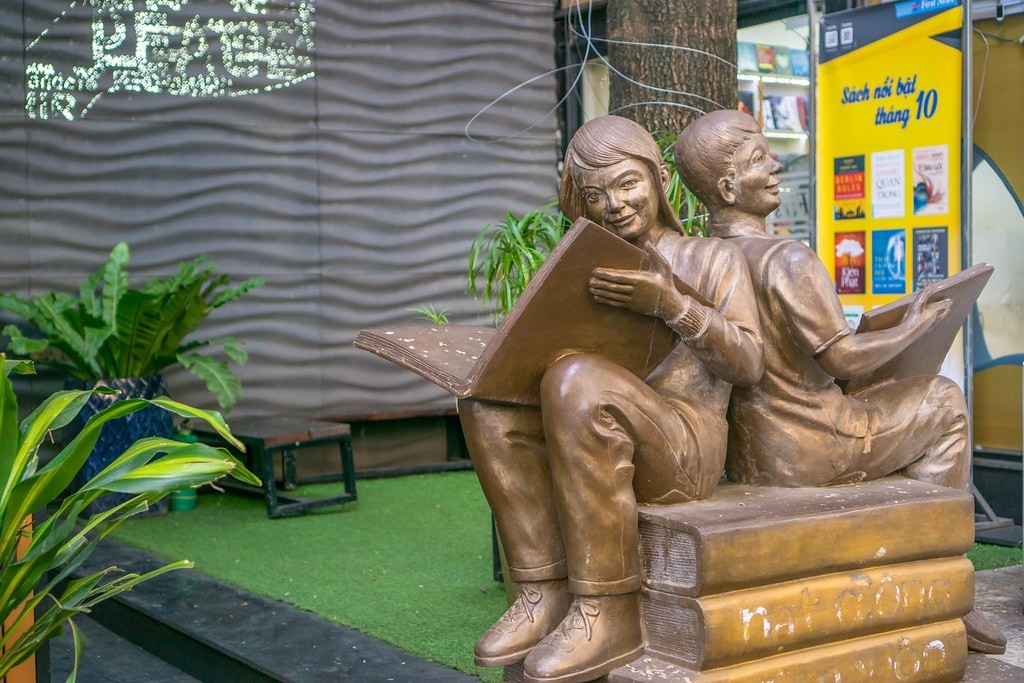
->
[186,417,357,517]
[277,403,474,485]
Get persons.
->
[676,108,1007,656]
[456,113,767,683]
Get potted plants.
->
[0,240,263,519]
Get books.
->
[597,471,978,683]
[738,41,810,79]
[738,84,811,238]
[833,260,995,397]
[355,216,713,411]
